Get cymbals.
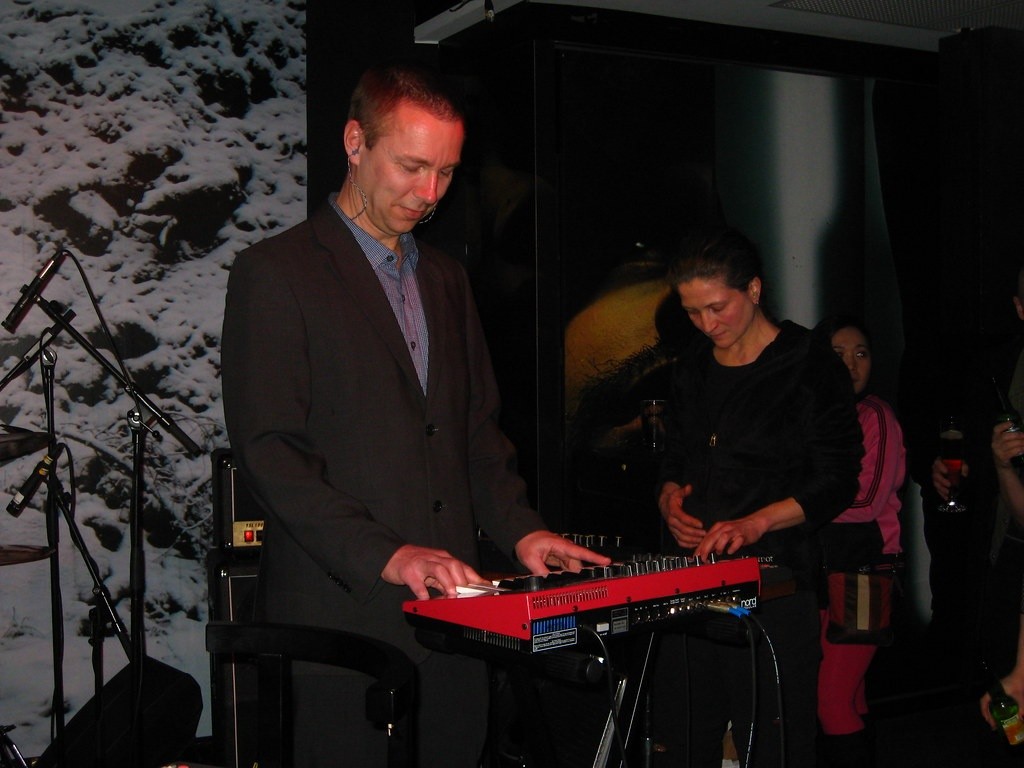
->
[0,544,58,568]
[0,422,56,466]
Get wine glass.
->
[937,414,968,514]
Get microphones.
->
[5,442,65,517]
[1,252,64,334]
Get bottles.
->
[989,371,1024,469]
[979,660,1024,747]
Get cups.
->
[641,398,666,458]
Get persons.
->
[219,68,613,768]
[933,261,1024,729]
[621,233,860,768]
[807,309,908,766]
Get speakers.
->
[206,549,293,768]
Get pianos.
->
[395,553,761,657]
